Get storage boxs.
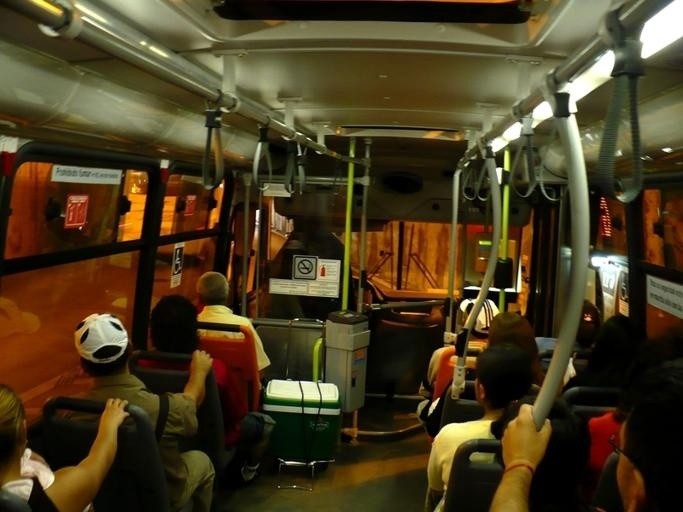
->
[325,311,370,413]
[263,380,341,478]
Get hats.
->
[460,298,500,335]
[74,312,129,363]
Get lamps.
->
[489,0,681,156]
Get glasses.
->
[610,433,640,469]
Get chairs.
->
[438,381,484,429]
[40,397,170,512]
[128,349,243,471]
[539,349,590,377]
[560,384,607,406]
[196,322,259,413]
[595,450,623,512]
[431,347,482,400]
[441,439,503,511]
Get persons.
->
[192,271,269,398]
[416,298,504,447]
[491,359,683,512]
[421,344,535,512]
[58,315,216,512]
[555,314,650,407]
[428,312,564,444]
[136,295,278,486]
[543,298,609,361]
[588,329,683,512]
[0,384,130,512]
